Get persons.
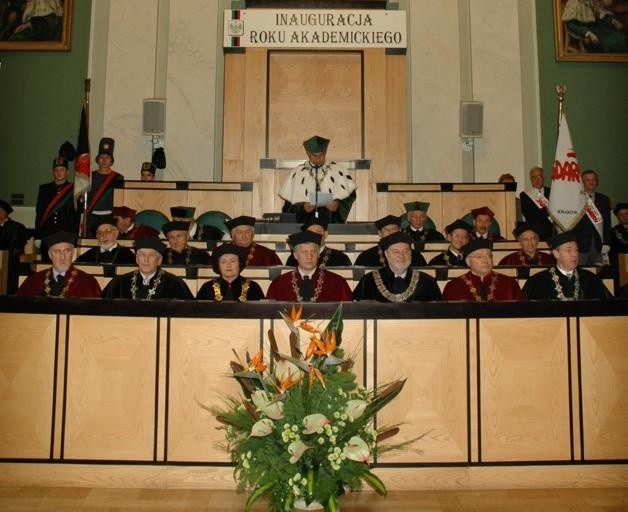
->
[141,163,156,181]
[499,174,522,224]
[612,203,628,298]
[574,169,612,267]
[101,237,195,300]
[197,216,283,301]
[74,206,213,266]
[80,154,124,239]
[352,202,554,300]
[519,167,552,241]
[16,232,102,300]
[267,223,354,301]
[0,200,31,296]
[279,136,358,223]
[562,0,627,53]
[523,234,613,301]
[35,158,84,264]
[4,0,64,42]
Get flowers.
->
[193,298,432,511]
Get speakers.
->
[142,99,164,133]
[459,102,485,139]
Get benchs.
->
[12,226,617,310]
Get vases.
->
[292,497,324,511]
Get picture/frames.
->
[551,0,627,65]
[0,0,78,52]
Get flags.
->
[73,100,93,213]
[546,112,588,233]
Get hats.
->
[404,202,430,211]
[95,138,114,164]
[289,219,328,246]
[135,237,167,256]
[212,245,245,275]
[472,207,494,219]
[375,215,412,250]
[225,216,255,231]
[53,159,68,170]
[614,202,628,215]
[45,232,76,247]
[512,223,540,238]
[162,207,196,233]
[549,231,578,248]
[142,162,155,175]
[445,219,493,256]
[0,199,13,214]
[94,206,136,229]
[303,135,329,153]
